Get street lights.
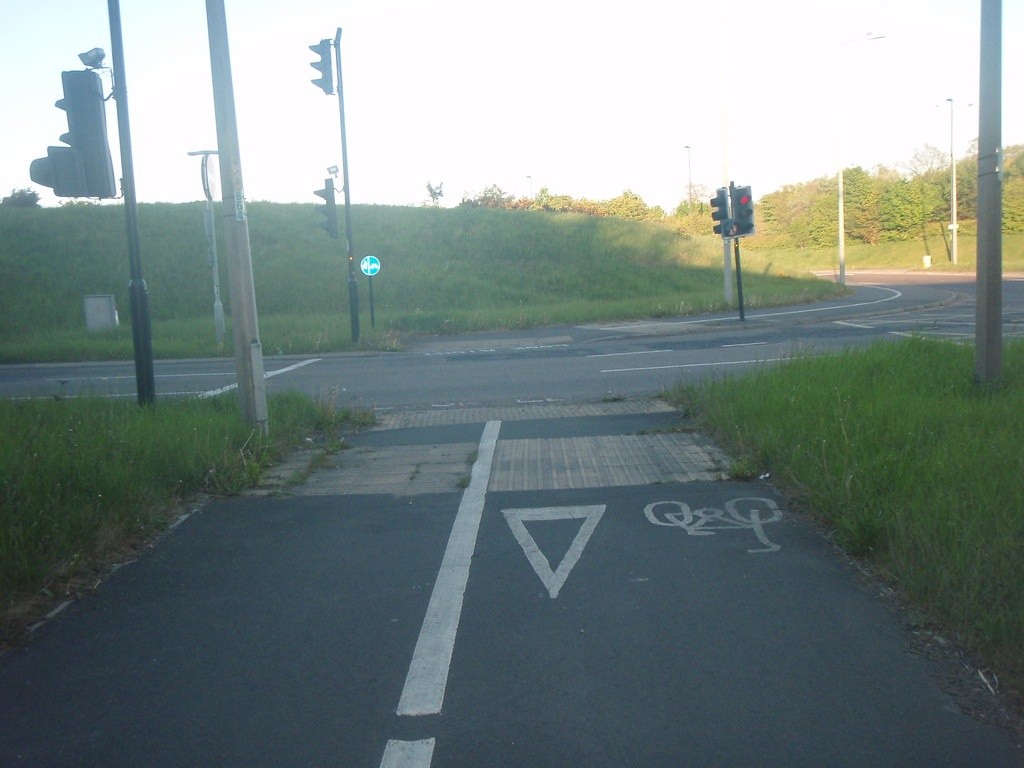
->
[684,144,697,216]
[833,29,888,284]
[186,149,223,348]
[945,96,961,260]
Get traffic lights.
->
[314,180,340,236]
[30,69,113,199]
[732,186,755,236]
[308,38,339,94]
[711,187,729,234]
